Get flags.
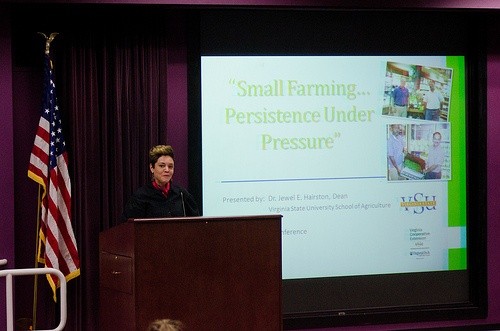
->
[27,57,83,302]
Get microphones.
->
[180,191,186,217]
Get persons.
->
[421,132,445,179]
[423,79,444,122]
[123,143,203,219]
[390,78,410,117]
[387,124,409,181]
